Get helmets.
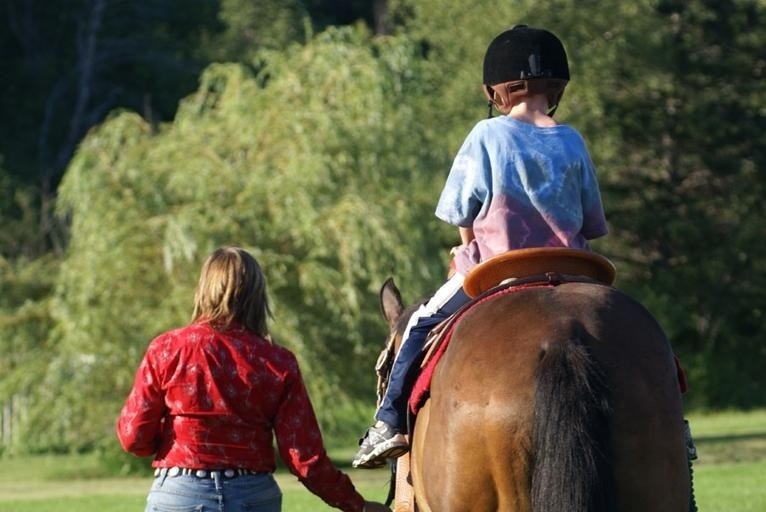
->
[483,24,570,86]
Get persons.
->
[114,246,389,512]
[351,22,609,469]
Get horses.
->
[381,277,699,512]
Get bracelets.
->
[363,500,367,511]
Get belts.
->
[153,468,258,480]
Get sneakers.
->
[352,426,410,469]
